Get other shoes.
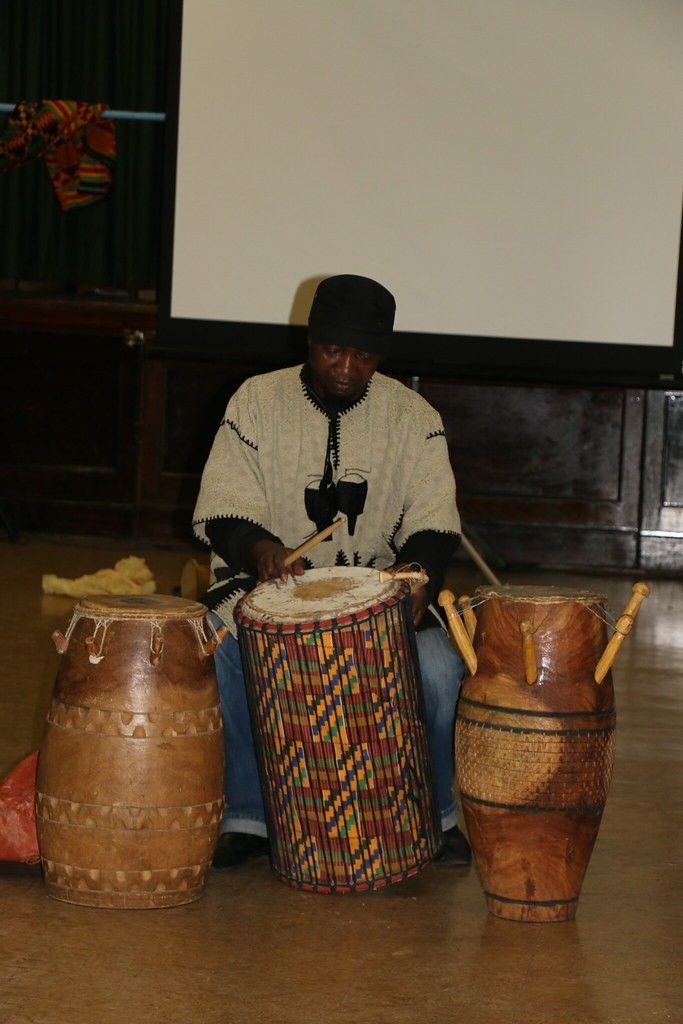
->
[434,823,472,867]
[212,826,266,871]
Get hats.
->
[308,274,397,355]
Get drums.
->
[32,589,233,911]
[229,562,446,896]
[437,581,651,925]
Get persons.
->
[192,275,475,870]
